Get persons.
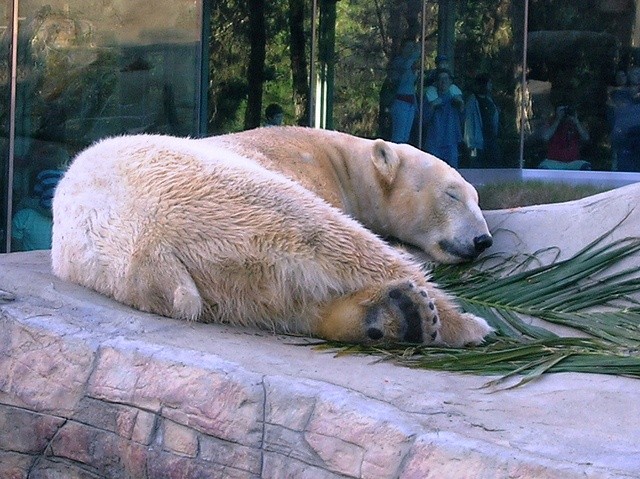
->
[378,62,393,141]
[605,70,640,107]
[391,39,422,143]
[427,56,451,86]
[423,69,465,169]
[263,103,285,129]
[467,73,500,166]
